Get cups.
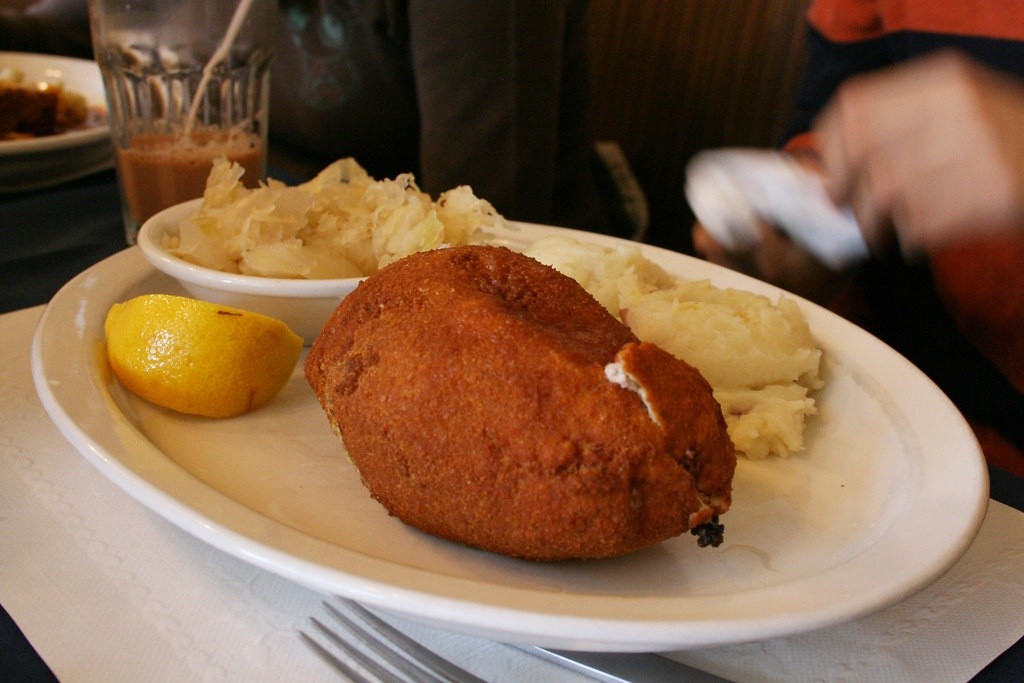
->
[88,0,275,247]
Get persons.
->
[0,0,1023,511]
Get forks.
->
[298,592,487,683]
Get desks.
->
[0,151,1024,682]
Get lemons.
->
[105,294,303,419]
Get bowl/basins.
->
[139,195,369,345]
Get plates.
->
[0,51,113,155]
[30,223,991,656]
